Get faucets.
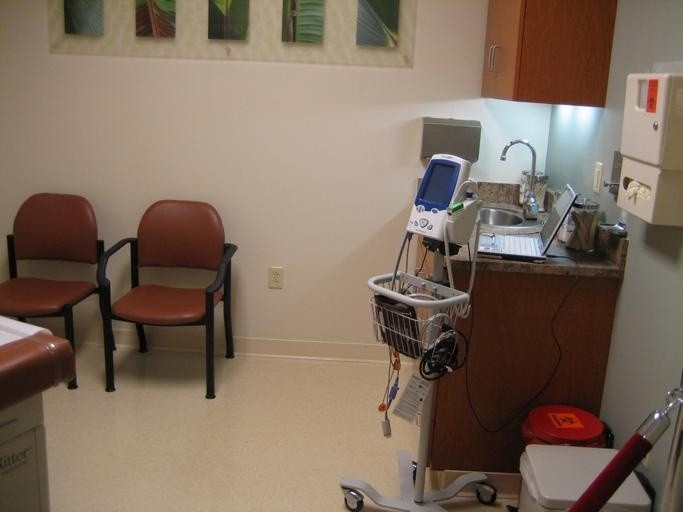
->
[499,138,537,193]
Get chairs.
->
[0,192,116,391]
[99,199,239,399]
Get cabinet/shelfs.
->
[479,0,618,108]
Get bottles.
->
[556,194,599,254]
[517,168,552,212]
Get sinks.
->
[480,206,545,230]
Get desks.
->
[0,318,79,512]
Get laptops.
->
[478,183,578,263]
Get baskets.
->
[368,271,470,358]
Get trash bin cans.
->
[521,405,615,449]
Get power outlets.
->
[268,266,283,290]
